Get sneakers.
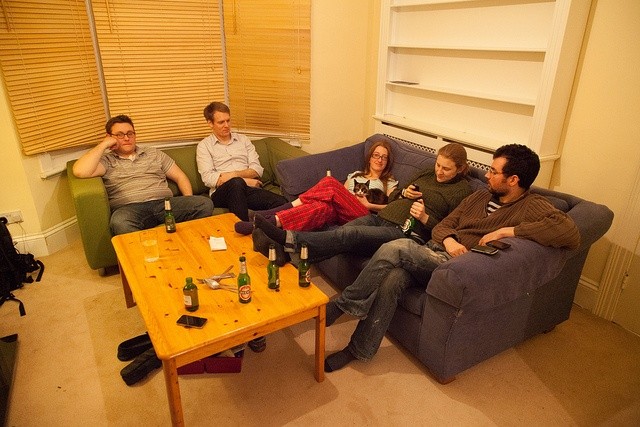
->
[244,336,266,352]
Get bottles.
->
[398,215,416,235]
[164,198,177,233]
[324,168,333,177]
[267,245,280,289]
[298,241,310,287]
[182,277,199,312]
[237,256,251,304]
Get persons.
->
[195,101,291,221]
[71,113,215,239]
[323,143,581,374]
[235,139,401,236]
[253,143,474,269]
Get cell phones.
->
[412,182,419,192]
[176,314,208,329]
[485,240,512,250]
[470,245,500,255]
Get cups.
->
[139,230,159,263]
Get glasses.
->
[371,153,388,160]
[489,167,508,175]
[110,131,135,139]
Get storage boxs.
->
[206,349,243,373]
[177,357,205,374]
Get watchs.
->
[401,187,407,199]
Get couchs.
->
[274,135,613,387]
[67,138,312,276]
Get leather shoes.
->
[118,332,152,361]
[121,347,162,386]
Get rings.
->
[413,210,417,214]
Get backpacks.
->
[0,218,33,316]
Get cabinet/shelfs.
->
[371,0,596,190]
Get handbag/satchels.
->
[17,254,44,281]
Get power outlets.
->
[2,209,24,224]
